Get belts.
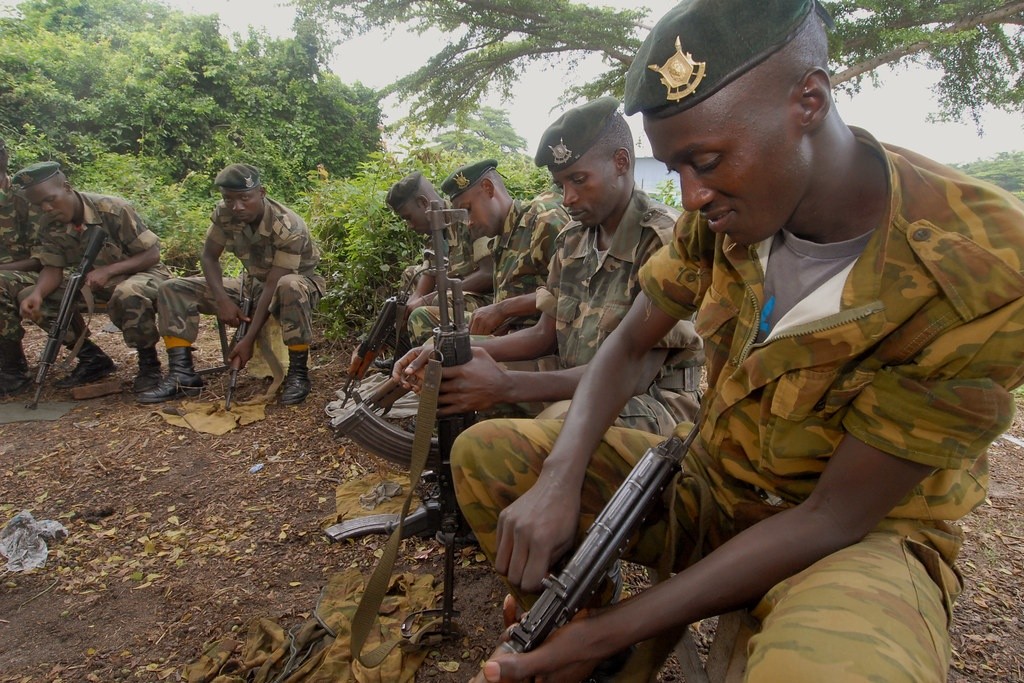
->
[657,365,700,392]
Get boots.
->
[281,348,311,405]
[54,338,118,389]
[550,543,633,682]
[134,347,161,391]
[136,346,206,404]
[0,339,33,399]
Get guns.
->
[339,291,411,411]
[224,269,258,413]
[29,225,107,414]
[324,198,501,545]
[507,430,692,650]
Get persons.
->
[11,161,172,393]
[451,0,1024,683]
[372,157,572,421]
[392,97,705,548]
[136,164,325,404]
[0,137,43,394]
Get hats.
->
[440,159,498,202]
[624,1,816,121]
[11,161,61,189]
[385,171,423,213]
[214,164,261,192]
[534,97,620,172]
